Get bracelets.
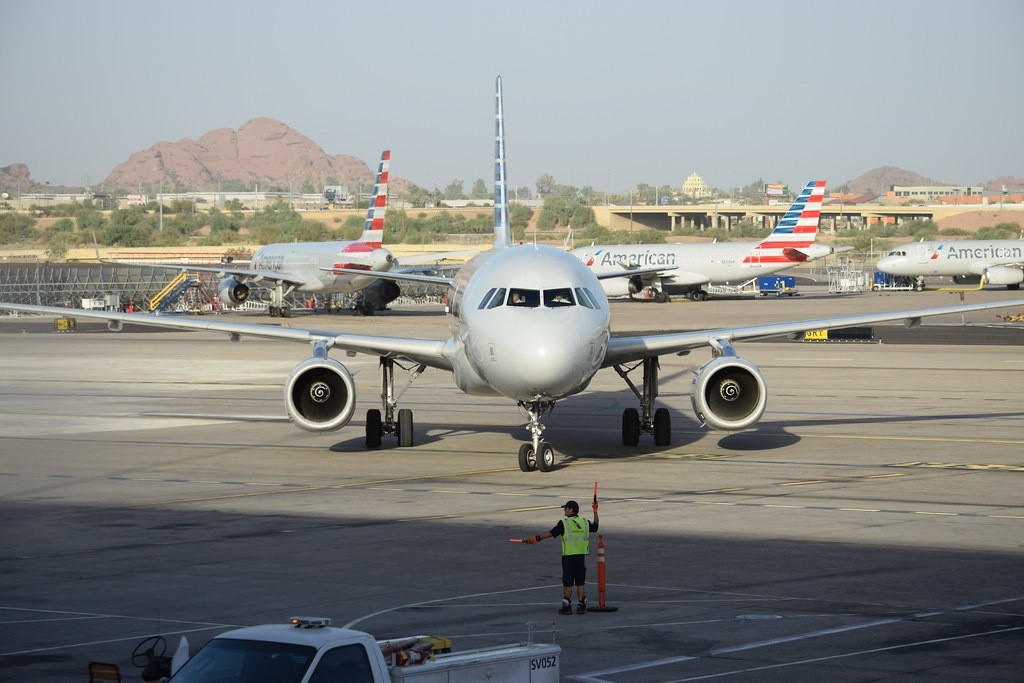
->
[535,535,542,541]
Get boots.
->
[559,596,572,614]
[577,596,587,613]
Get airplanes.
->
[92,150,482,318]
[0,75,1024,473]
[569,181,855,303]
[876,239,1024,292]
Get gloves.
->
[592,501,598,512]
[527,535,541,544]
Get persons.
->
[552,293,571,303]
[510,293,525,303]
[527,501,599,615]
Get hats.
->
[561,501,579,509]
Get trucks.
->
[168,615,561,683]
